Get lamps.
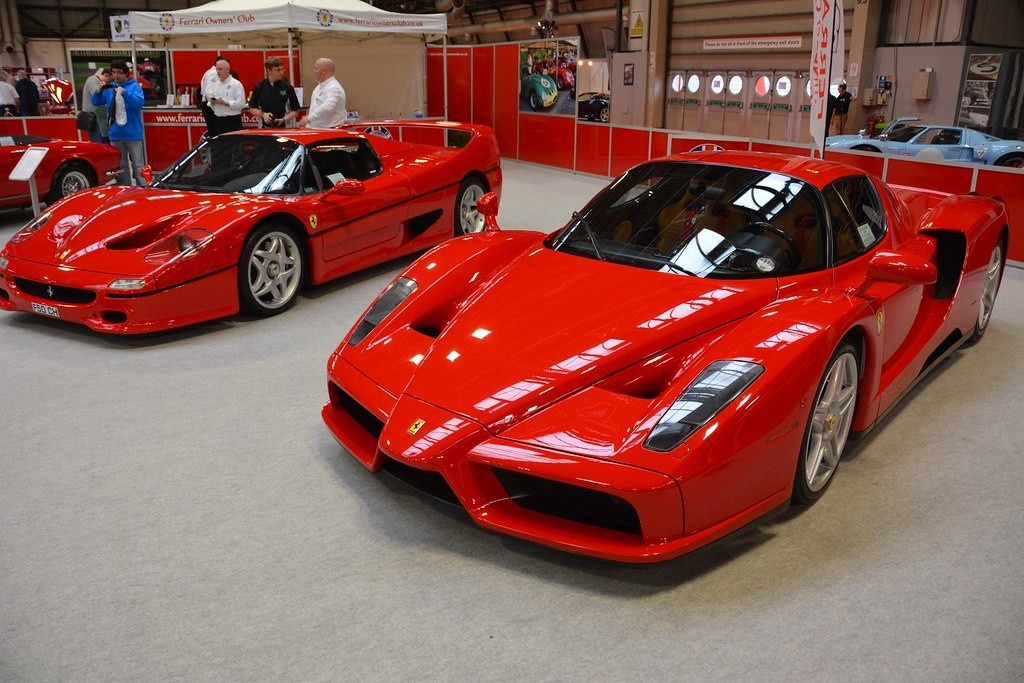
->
[536,18,557,39]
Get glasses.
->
[273,68,285,72]
[113,71,125,75]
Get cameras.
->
[269,115,278,127]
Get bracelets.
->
[283,117,287,123]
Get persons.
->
[831,83,859,135]
[90,61,146,187]
[206,60,246,172]
[247,56,302,129]
[200,56,239,126]
[298,58,347,129]
[82,68,114,144]
[13,69,40,116]
[0,70,20,116]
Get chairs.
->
[653,178,743,259]
[315,149,362,188]
[768,182,859,272]
[246,142,285,173]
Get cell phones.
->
[212,98,217,100]
[103,84,115,89]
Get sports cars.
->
[322,148,1012,564]
[0,134,123,209]
[0,119,502,335]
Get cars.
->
[824,117,1024,168]
[520,43,578,111]
[577,93,610,122]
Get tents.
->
[127,0,450,167]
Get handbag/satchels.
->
[76,111,97,131]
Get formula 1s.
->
[126,58,165,100]
